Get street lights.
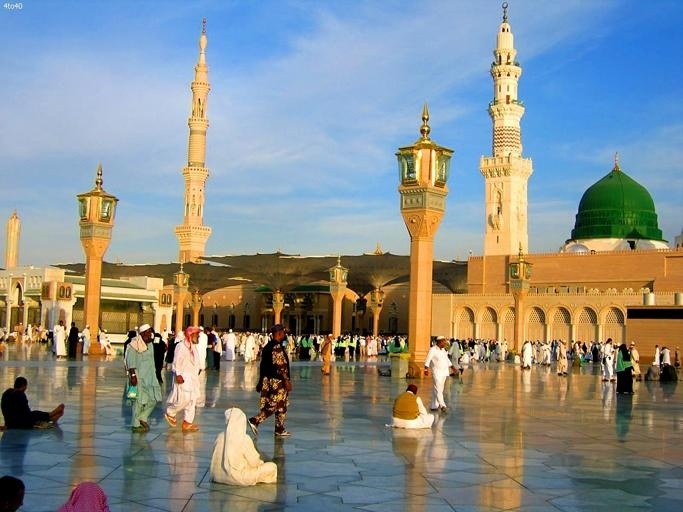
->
[272,287,285,327]
[390,104,456,381]
[75,163,119,354]
[170,261,189,333]
[189,288,201,329]
[508,241,533,357]
[68,357,100,502]
[271,402,286,484]
[325,364,345,445]
[506,366,527,463]
[367,283,385,336]
[324,252,350,340]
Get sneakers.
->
[275,430,292,437]
[247,418,258,436]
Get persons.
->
[431,336,508,376]
[590,338,642,395]
[606,436,634,511]
[122,324,222,434]
[336,332,408,363]
[54,479,110,511]
[207,406,278,486]
[521,339,587,375]
[220,330,335,376]
[0,432,31,477]
[1,377,65,429]
[393,384,435,428]
[0,322,49,355]
[52,320,91,362]
[646,345,681,384]
[206,486,278,512]
[98,327,111,355]
[247,325,292,436]
[390,435,426,471]
[164,436,199,511]
[423,336,457,413]
[426,437,449,484]
[0,474,26,511]
[123,434,158,512]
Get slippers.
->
[164,413,177,428]
[182,423,198,430]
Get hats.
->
[436,335,445,340]
[138,323,151,334]
[269,324,287,333]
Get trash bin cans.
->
[389,352,411,378]
